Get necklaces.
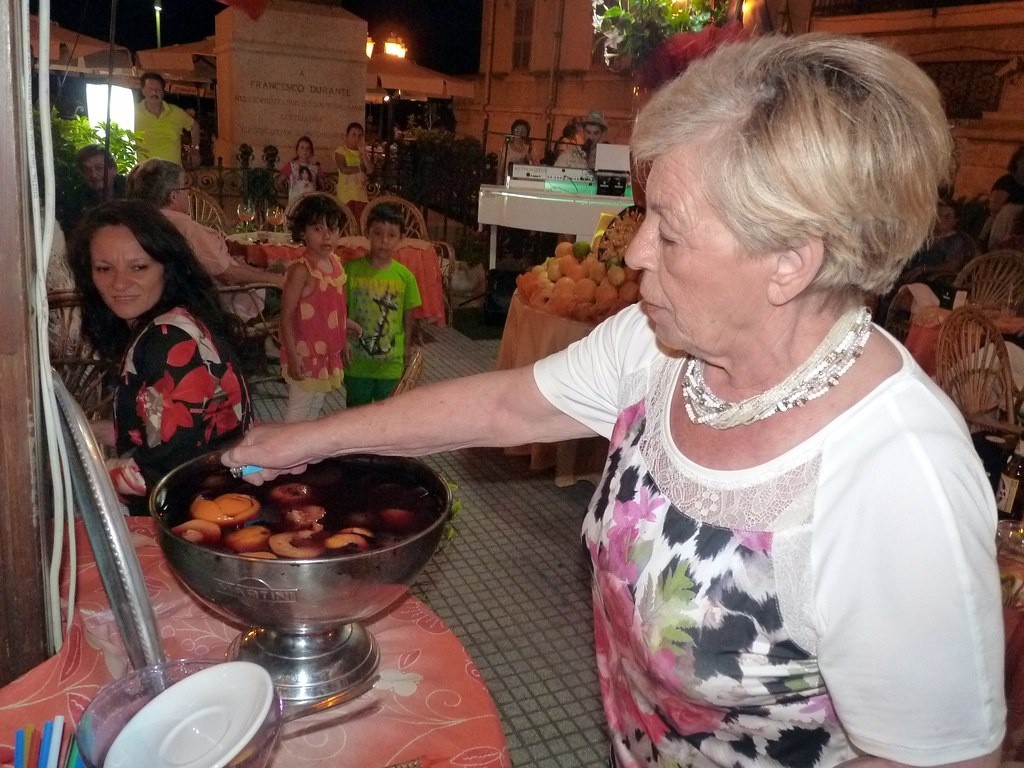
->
[681,305,871,430]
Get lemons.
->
[530,240,637,306]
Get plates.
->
[598,205,646,262]
[102,660,273,768]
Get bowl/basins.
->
[149,444,452,632]
[75,658,283,768]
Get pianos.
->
[477,183,637,288]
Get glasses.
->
[172,186,193,194]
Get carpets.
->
[453,306,508,340]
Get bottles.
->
[980,434,1024,522]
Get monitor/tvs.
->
[595,143,631,172]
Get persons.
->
[276,135,326,202]
[344,203,422,409]
[222,33,1008,768]
[135,72,200,170]
[71,144,127,205]
[498,110,607,184]
[335,122,374,205]
[281,194,362,423]
[903,146,1024,290]
[125,158,283,326]
[66,200,254,517]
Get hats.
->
[583,109,608,133]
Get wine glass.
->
[237,204,256,241]
[266,208,285,241]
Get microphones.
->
[579,137,593,158]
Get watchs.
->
[191,144,199,150]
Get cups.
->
[971,430,1019,496]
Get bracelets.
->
[922,264,927,274]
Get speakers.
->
[484,269,528,327]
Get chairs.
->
[47,187,455,462]
[884,229,1024,438]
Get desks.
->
[495,287,611,488]
[905,308,1024,377]
[224,231,448,351]
